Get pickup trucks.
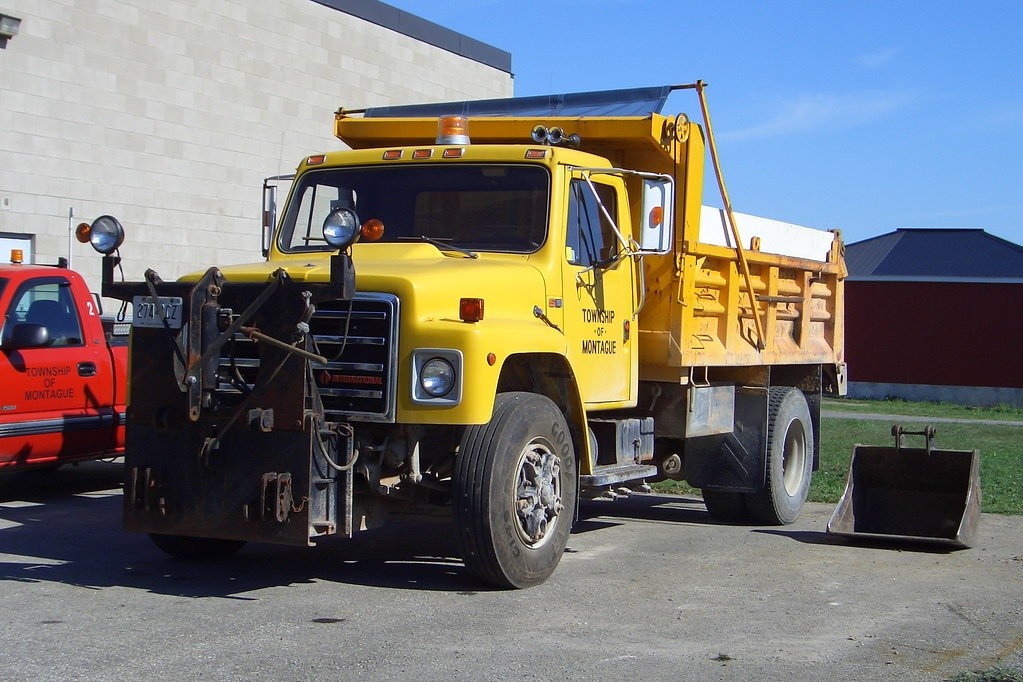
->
[0,262,132,475]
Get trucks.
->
[88,79,847,590]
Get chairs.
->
[26,299,66,339]
